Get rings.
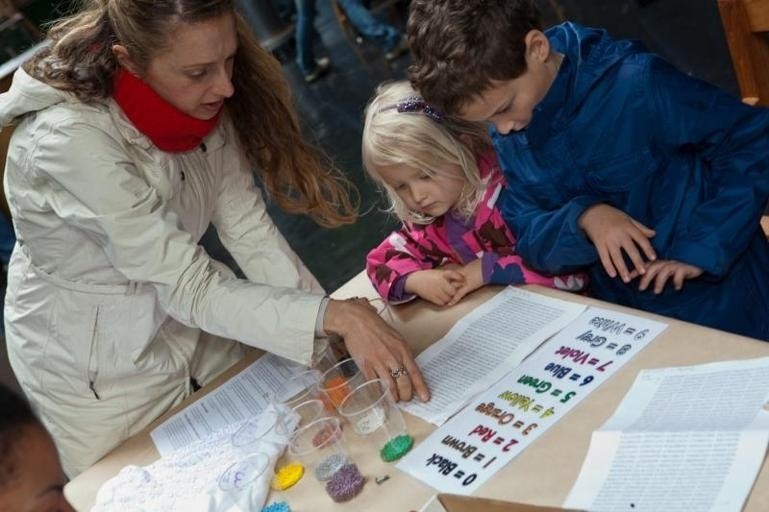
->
[391,366,407,378]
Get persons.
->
[2,2,431,480]
[361,81,591,307]
[294,1,415,83]
[405,1,767,343]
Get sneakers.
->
[384,33,415,62]
[306,56,333,85]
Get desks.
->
[62,270,769,512]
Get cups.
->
[230,409,303,489]
[288,417,366,503]
[217,454,292,512]
[273,369,343,448]
[338,379,414,461]
[318,358,391,438]
[275,399,349,482]
